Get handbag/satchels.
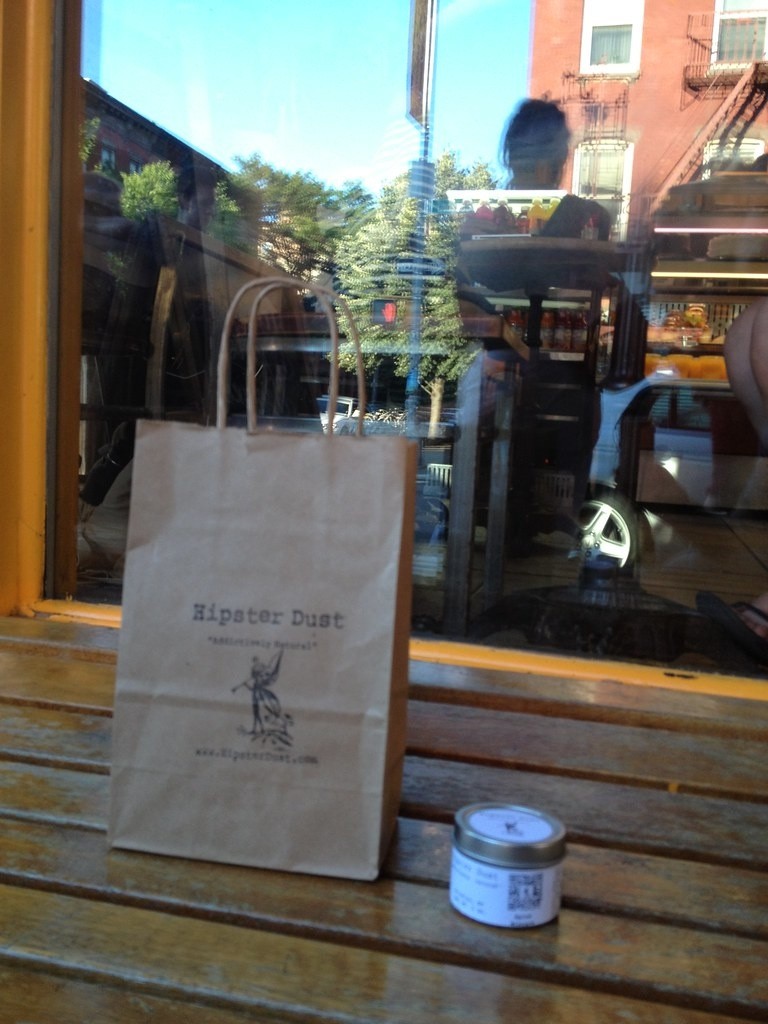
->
[110,277,419,884]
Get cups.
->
[645,325,727,380]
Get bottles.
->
[445,196,561,239]
[495,305,588,351]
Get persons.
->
[697,297,767,668]
[455,101,649,657]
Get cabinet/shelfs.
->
[232,314,531,645]
